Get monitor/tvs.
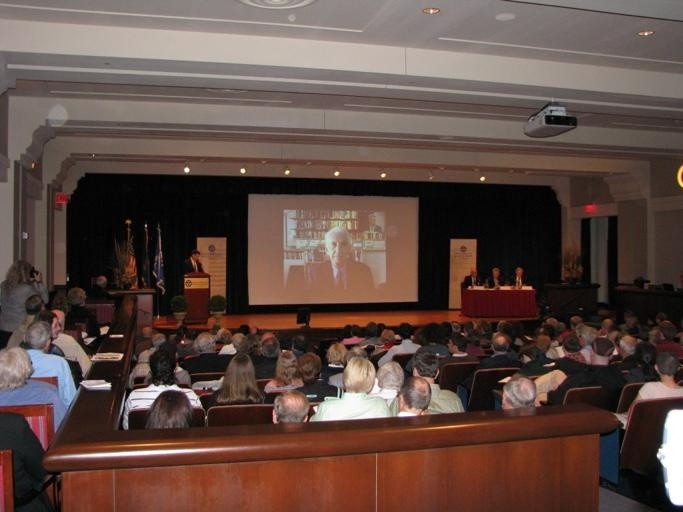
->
[663,283,675,292]
[297,306,311,328]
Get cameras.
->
[30,266,39,279]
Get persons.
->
[123,321,479,431]
[0,412,46,512]
[310,227,374,290]
[89,276,114,300]
[464,267,483,288]
[510,267,532,285]
[183,251,204,273]
[26,320,76,407]
[369,215,382,232]
[656,409,683,510]
[485,267,505,288]
[0,347,67,430]
[471,312,683,432]
[1,261,100,379]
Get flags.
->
[152,232,165,296]
[142,231,150,289]
[126,229,138,288]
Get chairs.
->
[0,300,683,512]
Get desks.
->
[615,286,683,325]
[462,288,537,317]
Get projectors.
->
[525,105,577,141]
